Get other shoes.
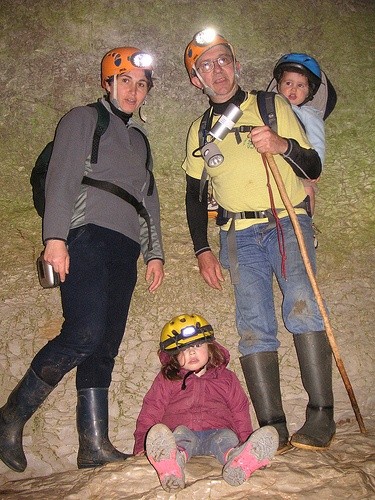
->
[144,423,185,493]
[222,421,279,486]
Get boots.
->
[0,368,59,473]
[289,330,336,452]
[239,351,288,453]
[76,386,136,469]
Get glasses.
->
[197,55,232,73]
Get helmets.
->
[160,314,215,351]
[101,46,154,88]
[272,53,322,88]
[184,32,235,79]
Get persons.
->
[273,52,325,183]
[0,45,165,472]
[182,29,335,456]
[132,315,280,494]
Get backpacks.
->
[29,103,151,218]
